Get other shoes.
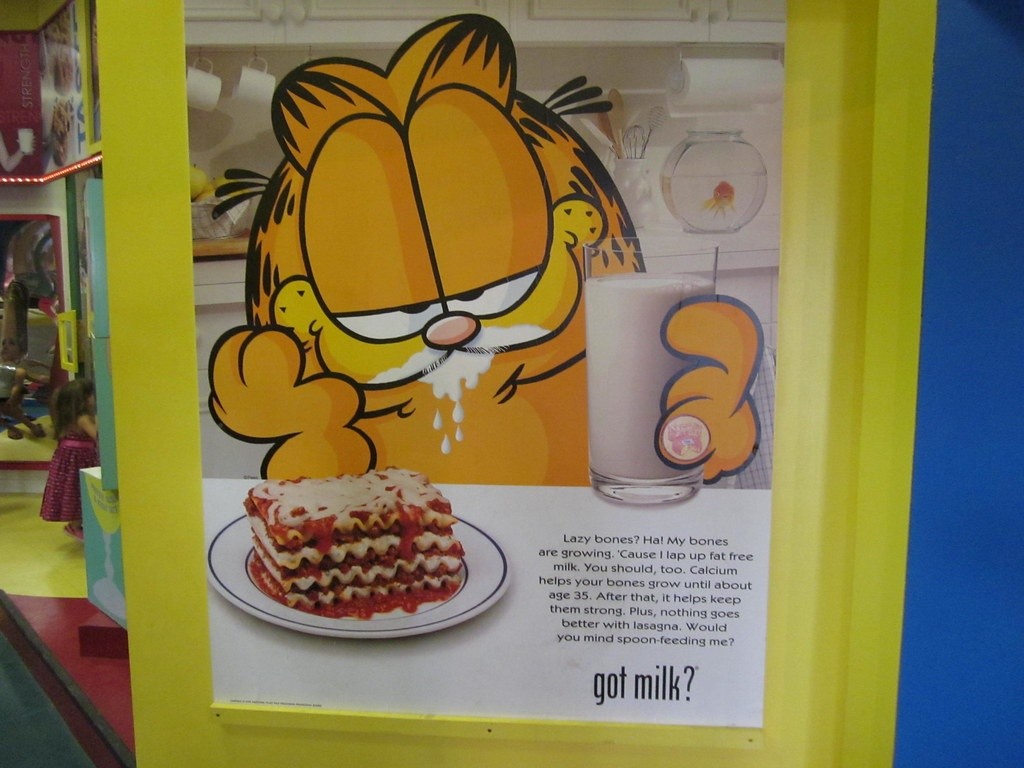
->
[64,524,85,543]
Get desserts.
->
[245,465,465,621]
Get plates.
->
[207,510,511,638]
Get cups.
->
[576,238,718,511]
[610,152,647,215]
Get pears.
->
[189,163,248,202]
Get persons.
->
[41,378,101,541]
